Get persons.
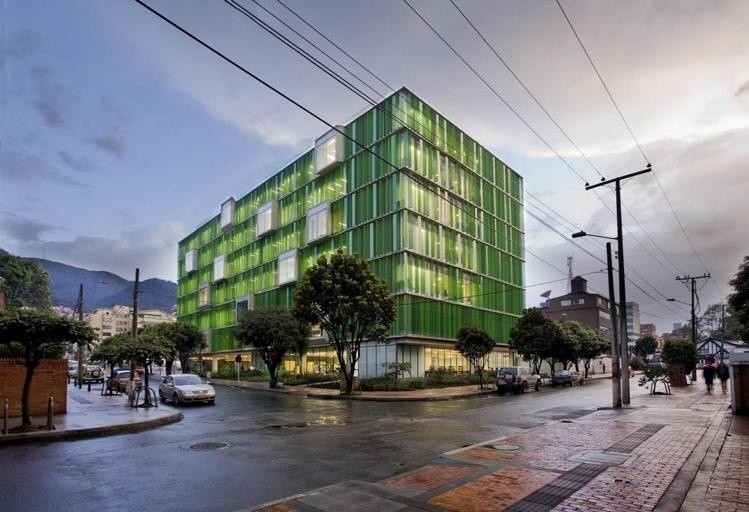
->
[716,358,729,392]
[703,361,716,394]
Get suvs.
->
[498,366,541,393]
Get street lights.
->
[568,230,635,408]
[667,298,697,381]
[129,312,162,390]
[78,326,101,389]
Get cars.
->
[552,370,585,388]
[67,365,146,392]
[647,362,665,368]
[158,373,216,406]
